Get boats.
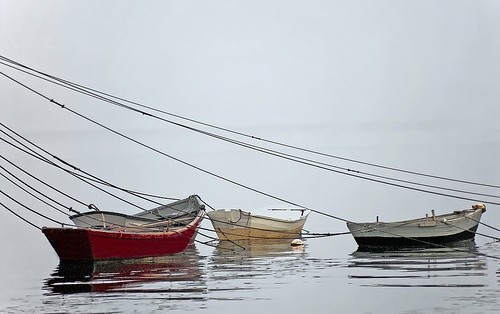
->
[345,199,486,252]
[41,203,206,263]
[206,208,312,251]
[68,192,204,244]
